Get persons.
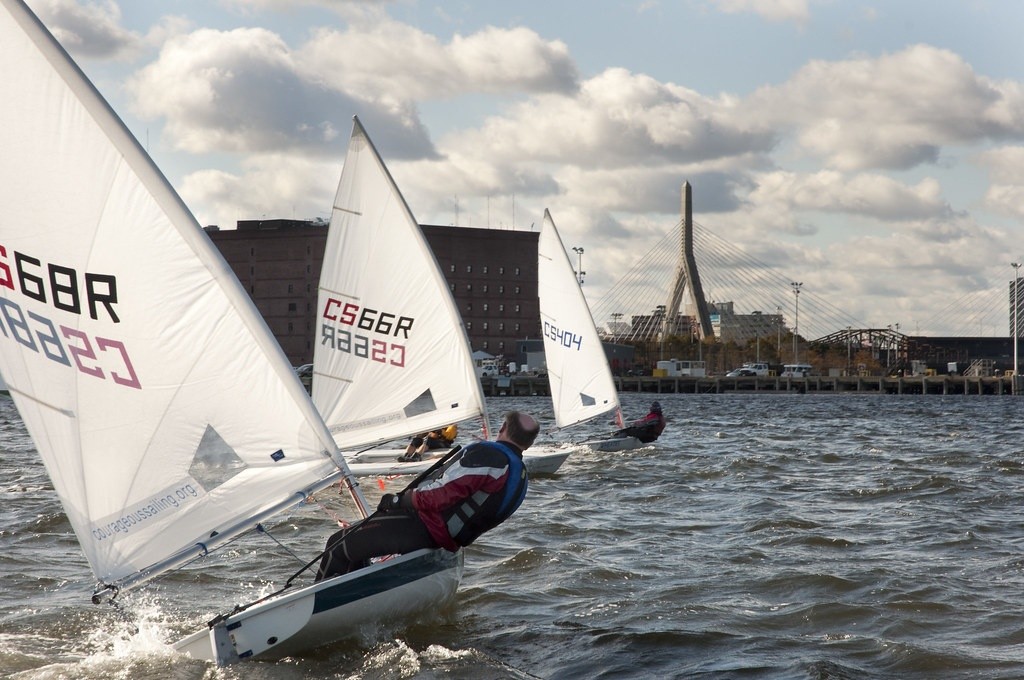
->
[609,398,666,446]
[311,411,542,588]
[476,350,659,389]
[395,423,459,464]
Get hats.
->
[649,400,662,412]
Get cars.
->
[295,364,314,380]
[481,359,515,377]
[726,368,752,377]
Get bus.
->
[780,365,812,377]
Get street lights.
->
[792,281,803,363]
[1011,261,1023,377]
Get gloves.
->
[376,492,404,512]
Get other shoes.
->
[398,452,422,462]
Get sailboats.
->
[536,207,653,456]
[306,113,573,485]
[1,0,463,669]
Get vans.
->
[745,364,769,377]
